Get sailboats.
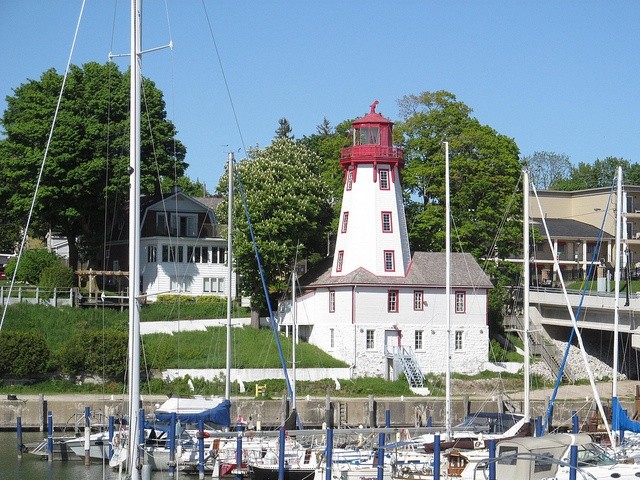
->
[26,141,640,480]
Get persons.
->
[320,421,327,446]
[246,417,254,442]
